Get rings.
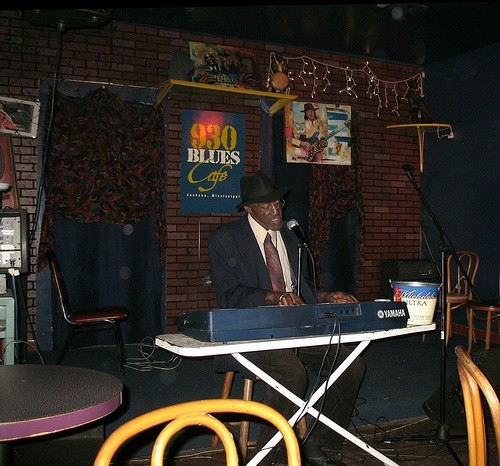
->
[279,295,284,301]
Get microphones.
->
[287,219,308,248]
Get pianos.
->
[172,299,411,345]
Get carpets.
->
[22,331,500,458]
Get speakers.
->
[381,260,436,301]
[422,387,466,426]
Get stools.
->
[211,356,310,461]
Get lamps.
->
[375,4,427,20]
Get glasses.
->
[248,198,285,216]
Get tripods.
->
[374,172,469,466]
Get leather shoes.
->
[302,451,348,466]
[270,459,288,466]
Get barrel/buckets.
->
[388,278,444,325]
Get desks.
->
[0,355,125,444]
[154,320,437,466]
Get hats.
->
[234,171,288,210]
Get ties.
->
[263,233,286,293]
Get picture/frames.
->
[282,99,353,167]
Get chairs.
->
[43,249,132,369]
[421,250,481,346]
[450,338,500,466]
[91,400,303,466]
[467,264,500,353]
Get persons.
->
[288,104,328,163]
[209,171,366,466]
